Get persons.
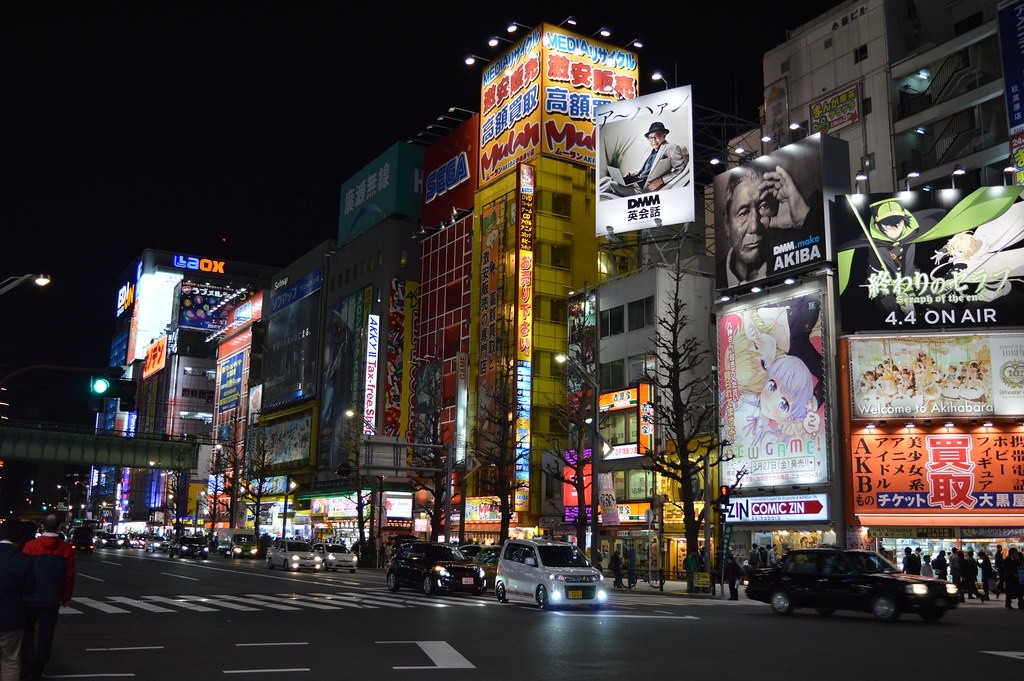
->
[682,545,742,600]
[452,534,549,546]
[717,154,826,289]
[902,547,949,580]
[379,541,392,569]
[878,547,888,569]
[275,536,347,547]
[599,122,685,196]
[161,433,203,443]
[600,494,616,508]
[597,549,603,573]
[948,544,1024,611]
[0,514,74,681]
[861,353,988,414]
[611,550,624,589]
[749,544,778,569]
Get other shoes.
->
[1005,605,1013,609]
[32,674,43,680]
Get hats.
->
[952,547,958,553]
[644,122,669,139]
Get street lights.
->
[346,409,379,436]
[556,353,599,569]
[149,461,168,535]
[215,444,240,528]
[57,485,70,523]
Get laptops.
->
[607,166,644,186]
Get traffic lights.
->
[89,375,138,397]
[720,486,729,504]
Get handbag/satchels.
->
[735,580,740,588]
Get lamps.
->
[488,35,515,46]
[437,115,463,123]
[652,72,669,90]
[441,221,448,230]
[508,22,535,33]
[558,15,577,27]
[410,228,421,240]
[465,53,489,66]
[448,107,475,116]
[625,38,644,49]
[417,131,444,137]
[854,165,1015,194]
[406,138,433,146]
[416,224,437,234]
[594,25,611,38]
[427,124,453,131]
[451,215,461,223]
[452,205,470,215]
[718,279,799,302]
[707,121,809,171]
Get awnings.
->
[286,477,367,500]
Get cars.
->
[229,534,259,559]
[744,547,961,623]
[146,536,171,553]
[460,545,503,587]
[387,541,488,597]
[312,543,358,572]
[67,527,150,553]
[169,537,209,560]
[266,540,323,572]
[495,539,606,611]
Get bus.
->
[70,518,100,529]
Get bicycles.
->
[621,564,666,588]
